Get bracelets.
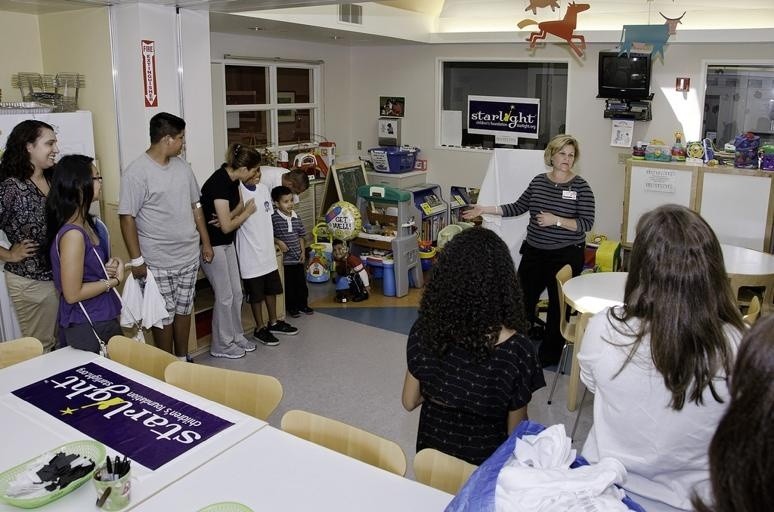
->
[495,206,498,215]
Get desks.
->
[131,425,455,510]
[2,346,269,510]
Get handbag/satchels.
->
[96,329,145,358]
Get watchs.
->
[557,216,561,227]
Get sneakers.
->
[267,319,299,335]
[253,326,280,345]
[291,307,313,317]
[210,346,246,358]
[234,337,257,351]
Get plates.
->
[198,501,255,512]
[0,439,107,508]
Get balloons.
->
[326,200,362,240]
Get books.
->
[422,217,445,242]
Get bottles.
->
[670,132,686,162]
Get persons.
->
[689,314,774,512]
[402,227,546,466]
[462,134,595,368]
[332,241,371,303]
[576,204,747,512]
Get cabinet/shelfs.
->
[188,244,286,357]
[405,183,448,246]
[366,170,426,190]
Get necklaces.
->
[554,184,558,188]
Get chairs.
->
[164,360,283,421]
[546,239,773,413]
[412,448,478,495]
[280,410,407,476]
[106,336,181,380]
[0,334,45,369]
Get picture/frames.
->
[276,90,297,125]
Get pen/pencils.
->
[99,452,131,480]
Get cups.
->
[92,462,130,510]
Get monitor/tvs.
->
[598,51,651,98]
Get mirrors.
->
[450,185,483,229]
